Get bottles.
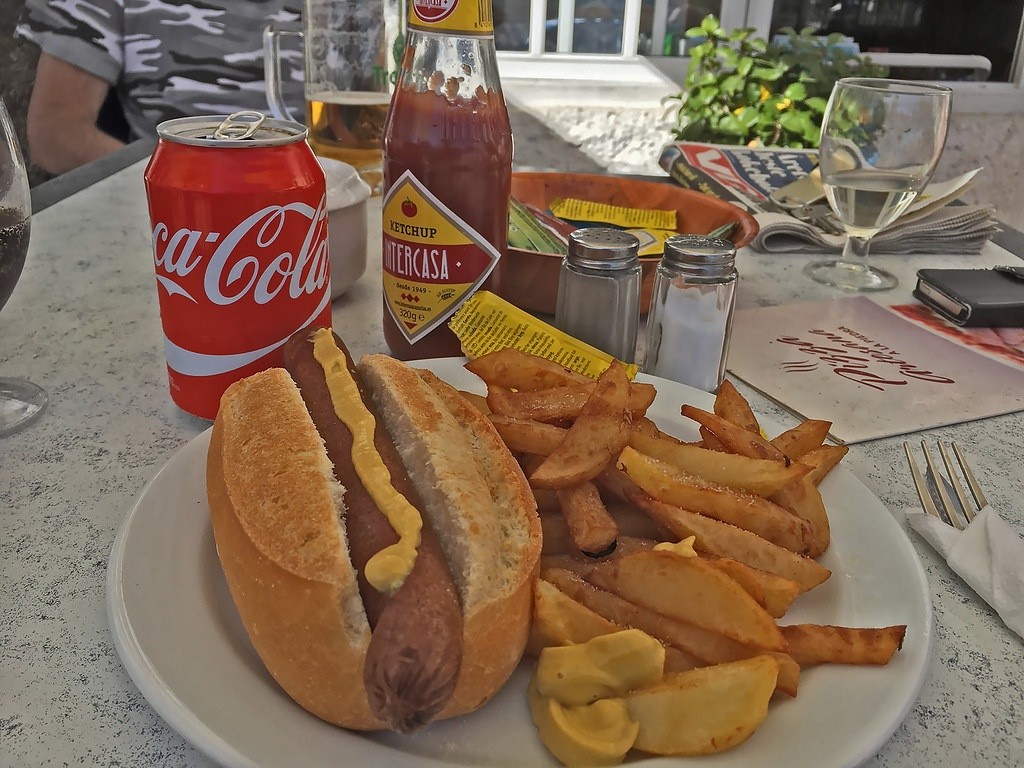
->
[376,0,513,361]
[555,225,643,366]
[641,233,739,395]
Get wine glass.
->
[804,75,955,296]
[2,101,52,438]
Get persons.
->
[9,0,310,176]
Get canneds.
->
[146,112,333,426]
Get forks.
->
[899,437,1024,638]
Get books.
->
[911,266,1024,327]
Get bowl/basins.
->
[310,153,374,305]
[505,165,762,318]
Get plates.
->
[106,352,939,768]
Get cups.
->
[262,1,406,199]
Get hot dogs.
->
[206,324,543,732]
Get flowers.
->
[667,16,888,159]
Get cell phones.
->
[916,268,1024,321]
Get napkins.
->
[910,508,1024,644]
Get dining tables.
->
[0,152,1024,768]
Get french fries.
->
[463,345,909,701]
